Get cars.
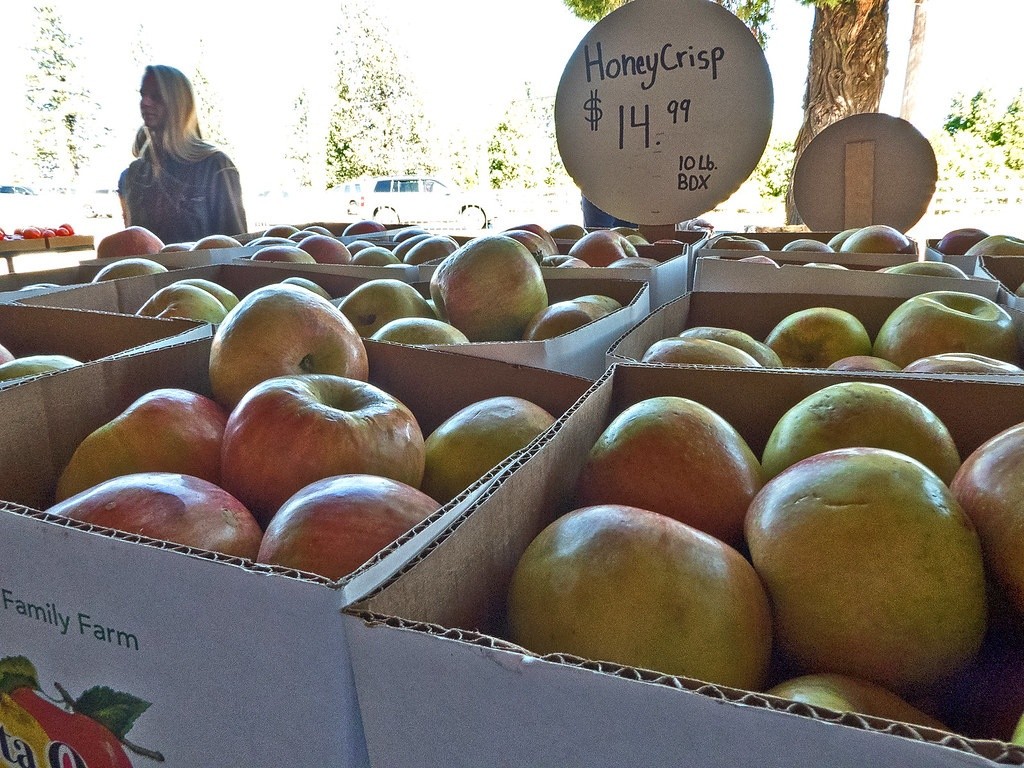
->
[0,185,38,197]
[81,185,123,219]
[357,175,499,229]
[331,181,361,216]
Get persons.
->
[581,194,714,231]
[118,64,249,244]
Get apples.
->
[1,219,686,583]
[505,224,1024,751]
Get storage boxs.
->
[0,222,1024,768]
